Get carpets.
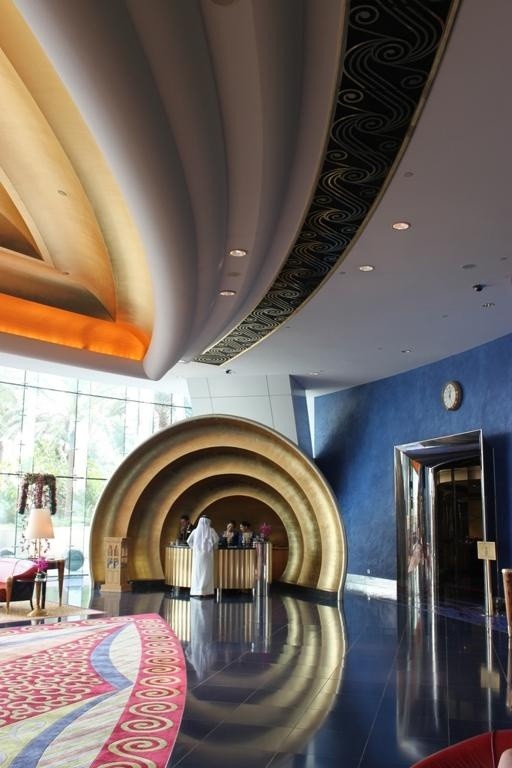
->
[0,613,188,768]
[0,601,105,624]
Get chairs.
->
[0,558,37,613]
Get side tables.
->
[30,557,65,609]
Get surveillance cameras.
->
[226,370,230,373]
[472,285,483,292]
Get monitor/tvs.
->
[218,537,228,549]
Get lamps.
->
[24,509,55,617]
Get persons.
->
[240,520,258,594]
[219,521,243,597]
[185,596,218,681]
[186,513,221,598]
[175,516,197,591]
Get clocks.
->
[443,382,462,409]
